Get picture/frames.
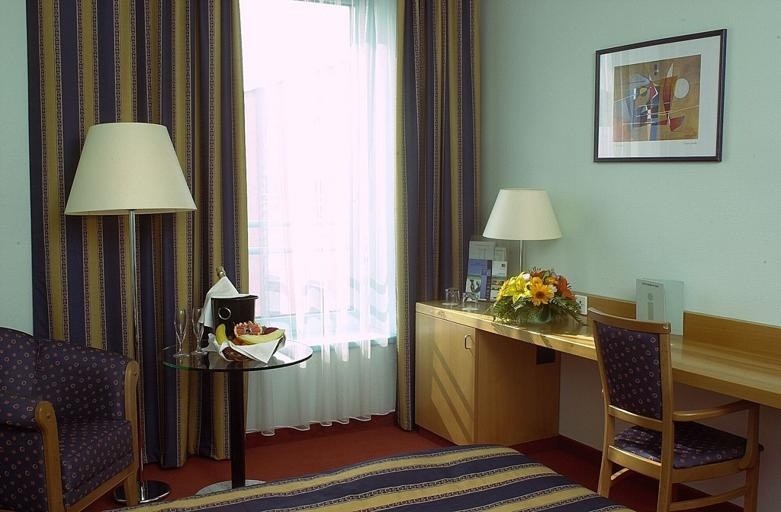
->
[592,27,727,163]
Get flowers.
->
[485,264,587,332]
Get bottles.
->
[215,265,226,280]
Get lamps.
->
[63,120,200,503]
[482,187,564,276]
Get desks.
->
[415,298,779,455]
[159,340,318,497]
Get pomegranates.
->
[234,319,261,337]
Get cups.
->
[445,286,479,309]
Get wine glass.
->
[189,307,208,356]
[173,306,188,360]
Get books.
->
[635,276,685,337]
[464,239,509,302]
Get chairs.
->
[584,305,768,511]
[1,325,145,512]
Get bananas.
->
[215,324,228,345]
[239,329,285,345]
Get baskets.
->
[223,337,285,362]
